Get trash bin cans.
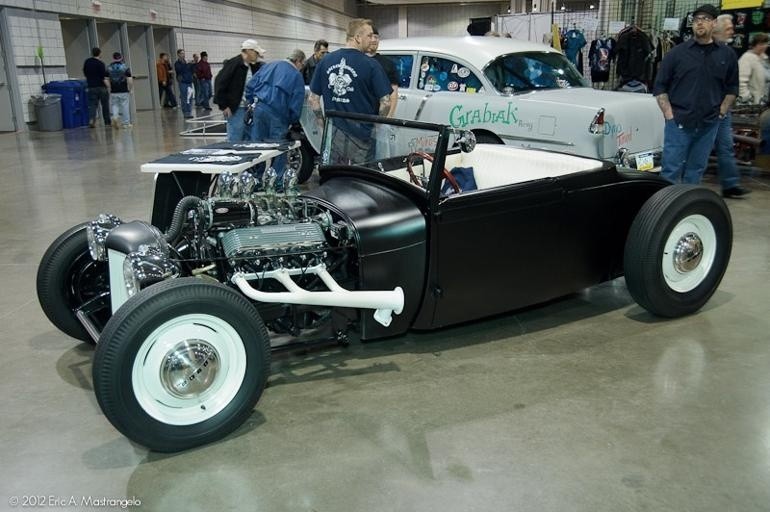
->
[41,80,88,129]
[32,94,63,132]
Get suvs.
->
[33,108,734,457]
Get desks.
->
[177,113,227,146]
[141,140,302,235]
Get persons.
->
[652,4,741,188]
[213,35,264,178]
[245,49,307,190]
[306,18,391,176]
[713,11,757,200]
[82,15,770,158]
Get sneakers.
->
[184,116,194,118]
[88,117,134,131]
[161,104,170,108]
[721,185,751,197]
[205,107,212,110]
[172,106,178,109]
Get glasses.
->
[320,51,330,54]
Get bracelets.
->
[719,110,724,116]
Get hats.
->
[692,4,718,18]
[241,39,266,54]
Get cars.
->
[280,32,669,185]
